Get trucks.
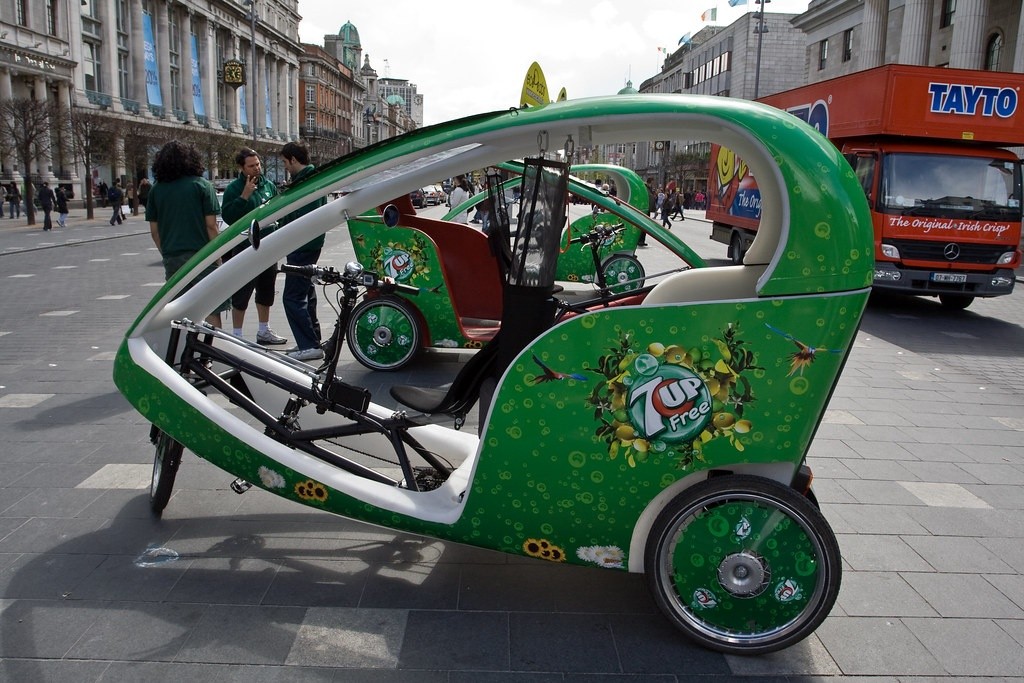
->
[705,62,1024,319]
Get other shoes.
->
[637,242,647,246]
[669,224,672,229]
[680,218,684,221]
[671,217,674,220]
[56,220,61,225]
[110,221,115,226]
[61,224,67,227]
[469,220,479,224]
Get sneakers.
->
[287,346,323,360]
[286,346,300,353]
[257,328,287,344]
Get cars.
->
[410,184,448,210]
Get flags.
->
[701,7,716,21]
[678,31,692,44]
[658,47,667,54]
[728,0,749,7]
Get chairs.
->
[388,161,568,414]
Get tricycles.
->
[110,89,878,659]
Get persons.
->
[636,178,657,246]
[221,146,287,345]
[0,177,38,219]
[38,182,57,231]
[282,142,327,360]
[448,172,520,230]
[144,137,232,330]
[53,183,69,227]
[571,174,708,230]
[97,177,152,225]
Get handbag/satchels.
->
[128,190,133,198]
